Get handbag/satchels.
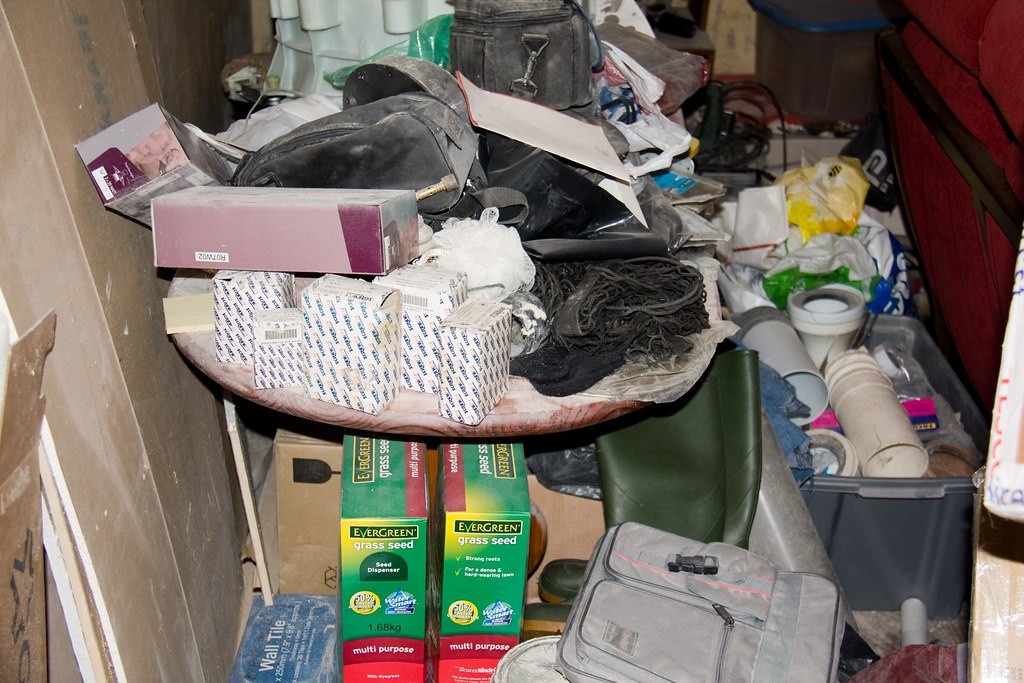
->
[449,0,594,109]
[556,521,845,683]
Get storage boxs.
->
[797,313,992,622]
[74,103,236,228]
[747,0,889,120]
[0,307,57,683]
[252,310,306,391]
[299,275,404,416]
[340,430,430,683]
[151,186,420,275]
[429,443,532,683]
[213,271,294,366]
[517,434,608,643]
[438,296,512,427]
[274,415,346,596]
[369,265,467,392]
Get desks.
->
[165,106,727,441]
